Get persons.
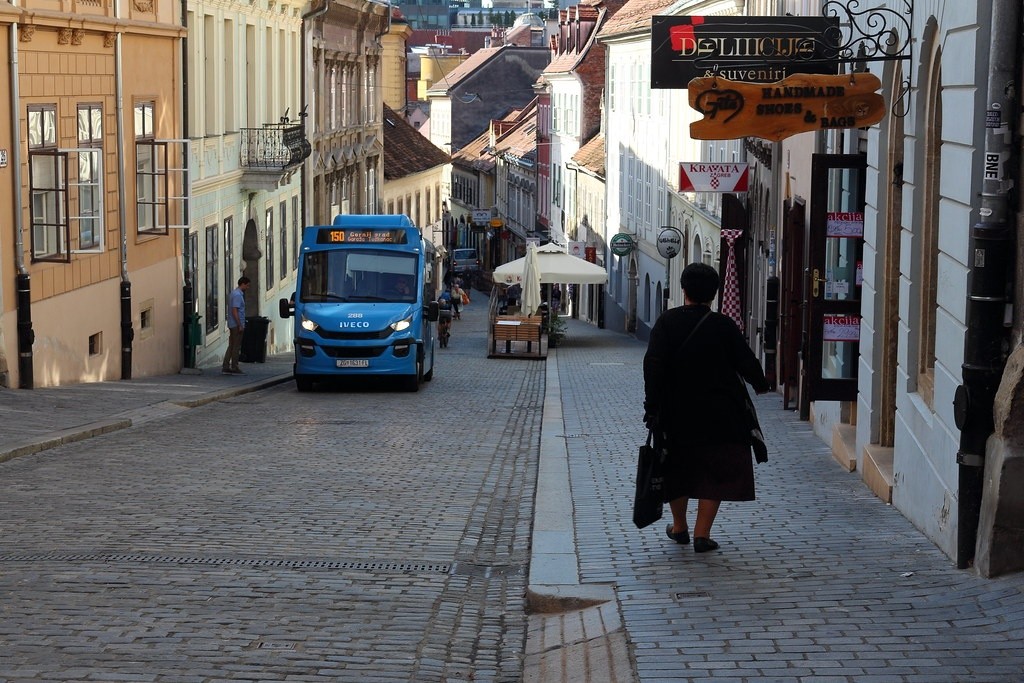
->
[631,262,769,554]
[505,285,519,306]
[438,267,472,340]
[552,285,562,317]
[221,278,250,376]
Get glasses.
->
[399,280,405,284]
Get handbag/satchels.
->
[633,429,663,529]
[751,428,769,463]
[458,302,463,312]
[463,294,469,305]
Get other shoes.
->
[233,371,248,375]
[694,537,718,552]
[666,523,690,544]
[222,368,232,374]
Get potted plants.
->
[548,313,568,348]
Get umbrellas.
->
[520,242,541,316]
[492,243,608,334]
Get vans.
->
[447,247,479,278]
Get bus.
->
[278,213,443,395]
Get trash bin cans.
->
[238,316,269,362]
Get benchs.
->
[493,315,543,356]
